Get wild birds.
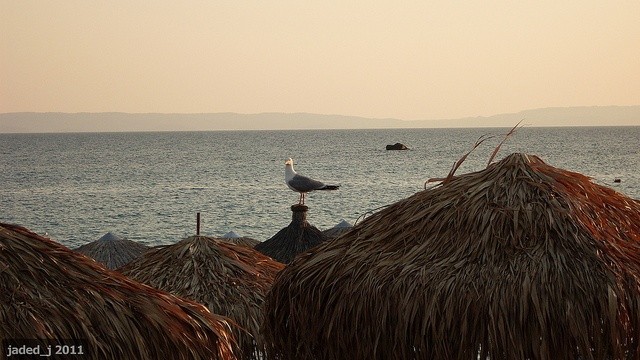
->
[285,157,339,207]
